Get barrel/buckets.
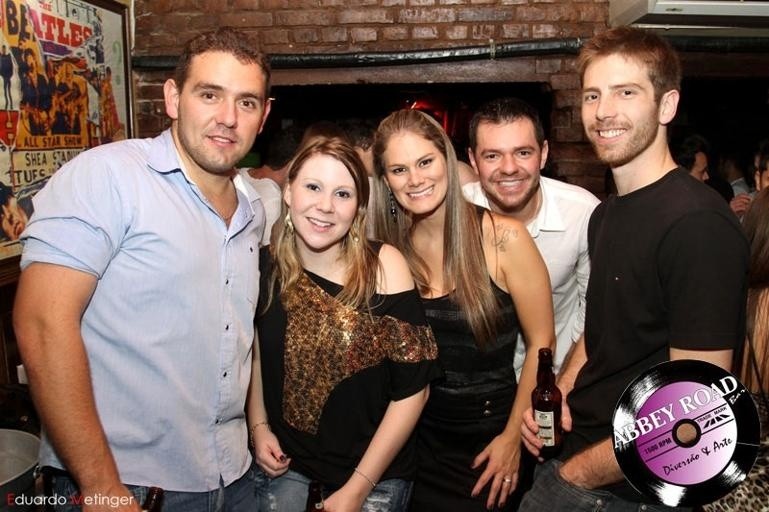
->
[0,429,43,512]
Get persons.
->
[239,27,769,511]
[12,27,277,512]
[0,45,13,110]
[0,181,34,240]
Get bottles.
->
[531,348,563,460]
[136,488,164,511]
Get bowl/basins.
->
[0,430,48,489]
